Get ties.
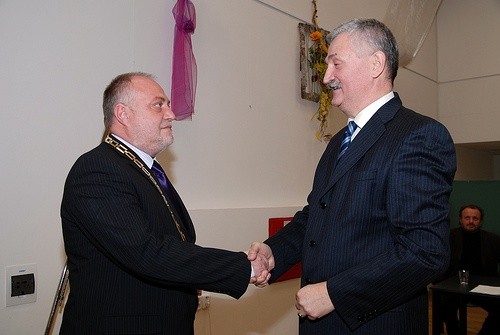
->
[331,120,357,179]
[152,160,196,244]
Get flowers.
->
[306,29,333,146]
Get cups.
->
[458,269,469,285]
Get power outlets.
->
[197,295,210,312]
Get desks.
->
[430,273,500,335]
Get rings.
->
[297,310,307,318]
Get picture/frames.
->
[298,22,331,102]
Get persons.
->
[59,72,269,335]
[247,17,457,335]
[432,203,500,335]
